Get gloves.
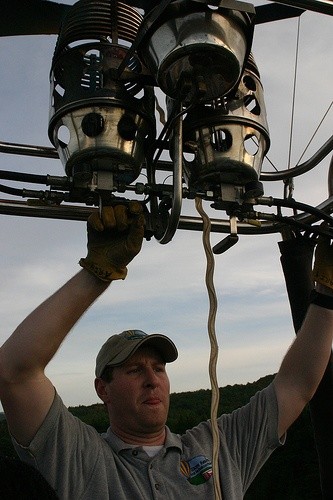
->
[78,202,146,281]
[309,235,333,290]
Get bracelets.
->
[308,288,333,310]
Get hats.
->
[95,330,178,379]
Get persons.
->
[0,199,333,500]
